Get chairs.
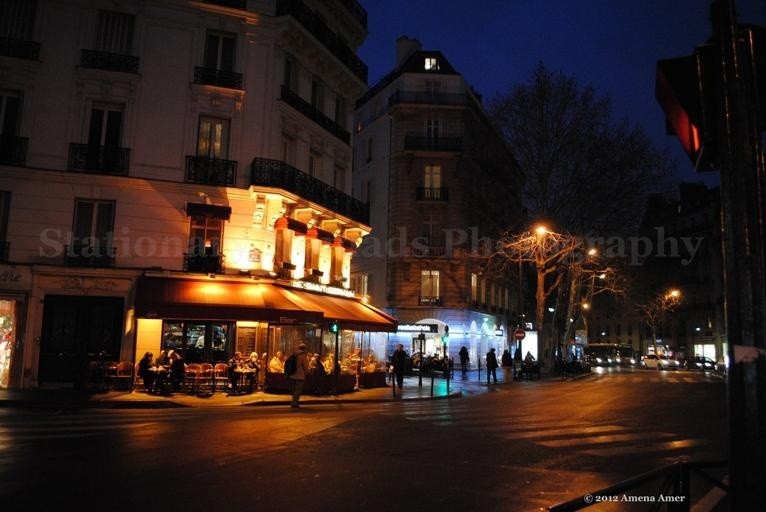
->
[263,353,386,396]
[80,361,261,395]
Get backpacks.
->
[285,352,304,376]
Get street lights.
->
[442,325,449,394]
[518,224,544,329]
[331,322,341,392]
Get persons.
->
[502,349,514,383]
[291,342,309,407]
[458,345,469,371]
[525,352,535,365]
[138,350,183,395]
[390,344,406,388]
[487,348,500,383]
[229,350,383,390]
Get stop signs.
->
[514,329,526,341]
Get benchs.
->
[417,356,454,378]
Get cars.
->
[639,355,680,371]
[684,356,717,370]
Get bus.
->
[587,342,635,367]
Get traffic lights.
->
[653,41,716,173]
